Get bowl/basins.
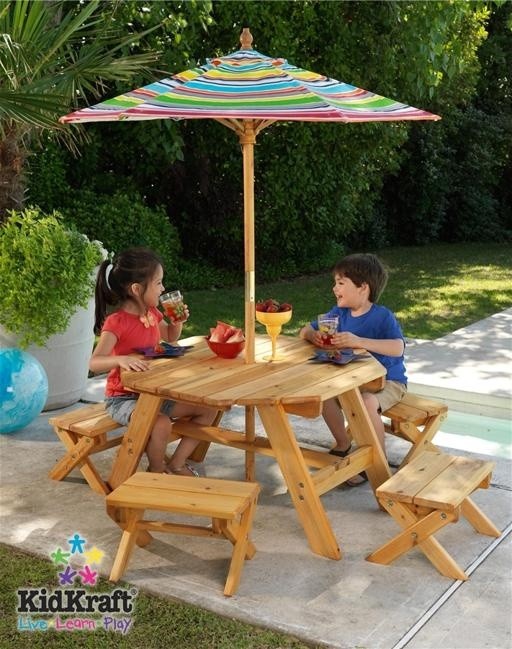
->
[205,336,247,360]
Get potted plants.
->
[0,203,116,412]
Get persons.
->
[86,246,220,477]
[297,252,407,487]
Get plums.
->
[256,298,293,312]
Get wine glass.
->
[254,309,294,362]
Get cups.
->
[157,288,189,325]
[316,312,344,360]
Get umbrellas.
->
[58,25,445,483]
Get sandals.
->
[329,443,369,487]
[145,463,200,478]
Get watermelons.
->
[210,320,245,343]
